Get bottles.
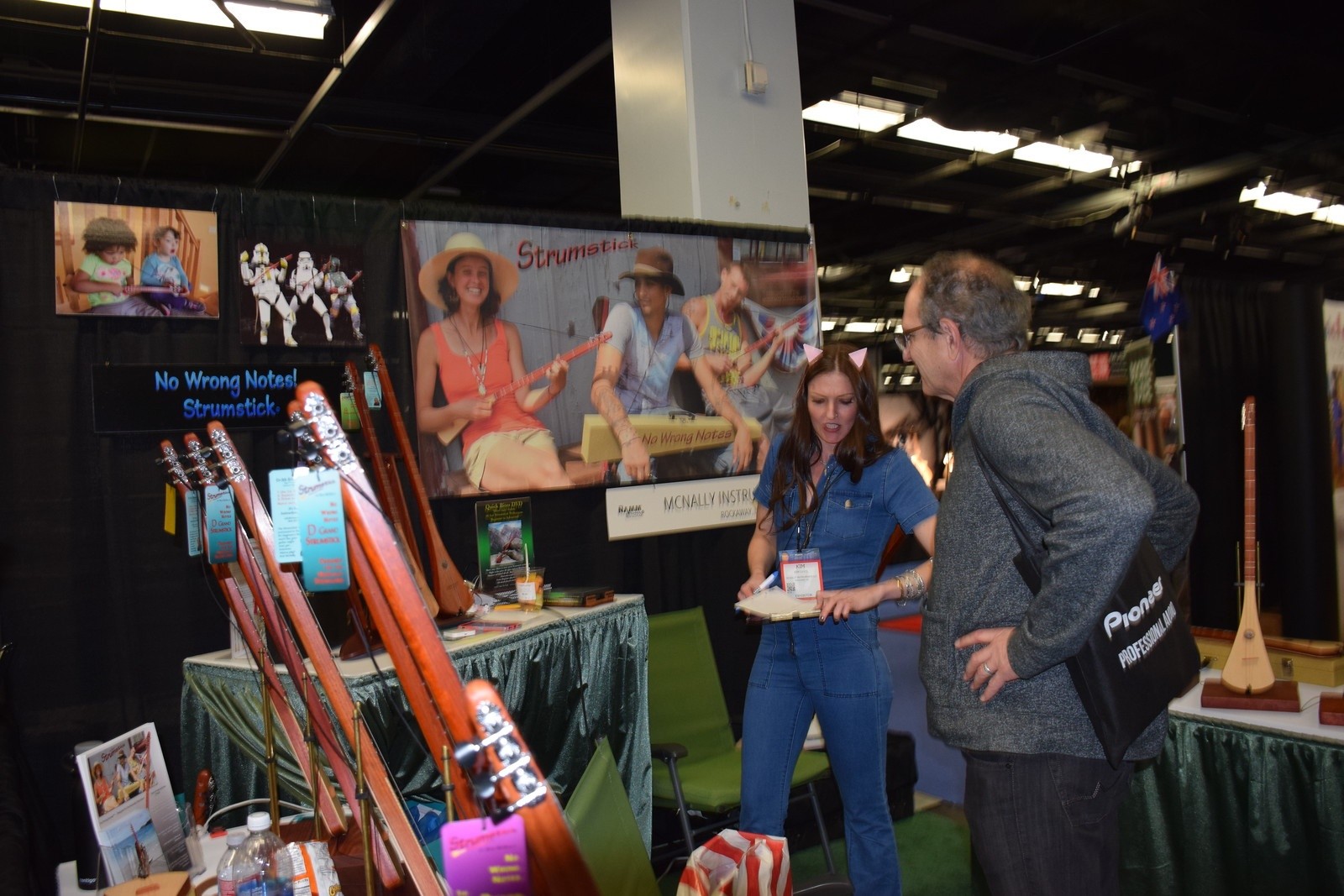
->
[175,794,206,878]
[514,567,545,613]
[216,811,294,896]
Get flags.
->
[1139,242,1180,339]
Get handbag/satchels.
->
[676,827,793,895]
[971,438,1201,770]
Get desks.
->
[180,583,654,867]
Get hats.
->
[617,247,685,296]
[416,231,518,312]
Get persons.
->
[735,343,941,896]
[92,747,151,811]
[414,233,571,490]
[589,248,755,486]
[141,226,206,316]
[672,260,783,436]
[897,252,1202,896]
[71,217,163,316]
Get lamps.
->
[1012,129,1117,179]
[893,111,1025,161]
[48,2,331,46]
[800,85,909,138]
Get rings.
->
[982,662,993,675]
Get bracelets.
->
[546,385,560,397]
[895,568,925,608]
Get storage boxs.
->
[1191,626,1344,689]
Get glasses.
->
[893,321,936,353]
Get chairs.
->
[640,604,842,896]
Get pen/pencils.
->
[494,604,520,609]
[735,571,778,611]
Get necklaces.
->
[449,309,487,373]
[452,317,490,398]
